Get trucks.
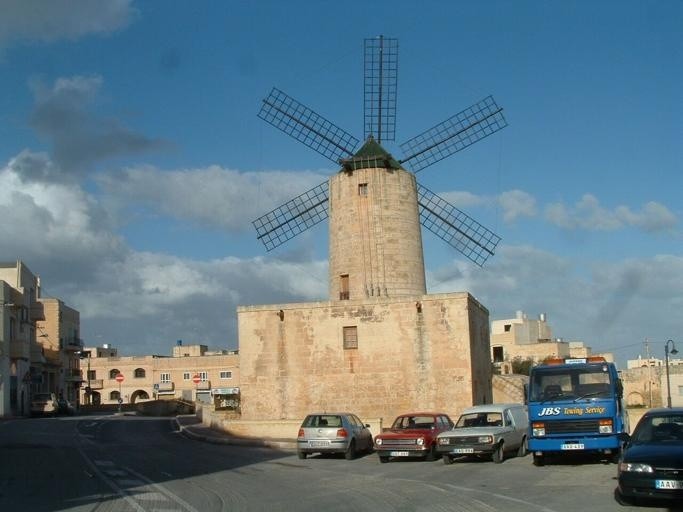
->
[520,355,629,467]
[434,401,530,467]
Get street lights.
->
[79,357,91,408]
[664,338,679,408]
[649,377,655,408]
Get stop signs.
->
[192,374,201,384]
[115,373,125,383]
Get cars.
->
[372,411,457,463]
[293,410,375,462]
[614,405,683,506]
[58,398,76,417]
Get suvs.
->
[28,390,60,417]
